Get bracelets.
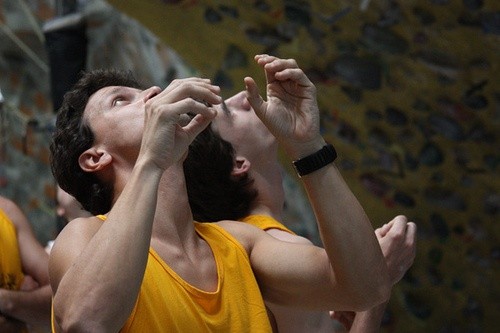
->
[291,143,337,179]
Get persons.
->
[47,53,393,332]
[184,89,419,333]
[42,179,97,257]
[0,193,54,333]
